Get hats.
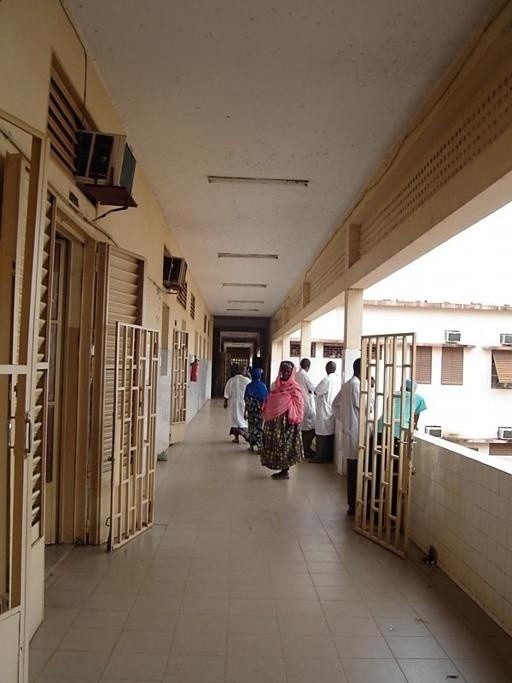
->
[250,368,261,379]
[405,380,417,393]
[282,361,293,368]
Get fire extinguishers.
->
[190,356,200,382]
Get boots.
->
[233,439,240,443]
[247,447,253,451]
[304,449,327,462]
[347,505,355,515]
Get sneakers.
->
[272,471,289,480]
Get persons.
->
[293,357,316,458]
[338,357,373,516]
[307,360,337,462]
[254,360,307,480]
[368,378,426,535]
[222,364,250,442]
[243,368,268,453]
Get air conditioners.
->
[444,329,461,343]
[500,333,512,346]
[424,424,443,439]
[497,426,512,440]
[162,255,189,290]
[75,128,139,197]
